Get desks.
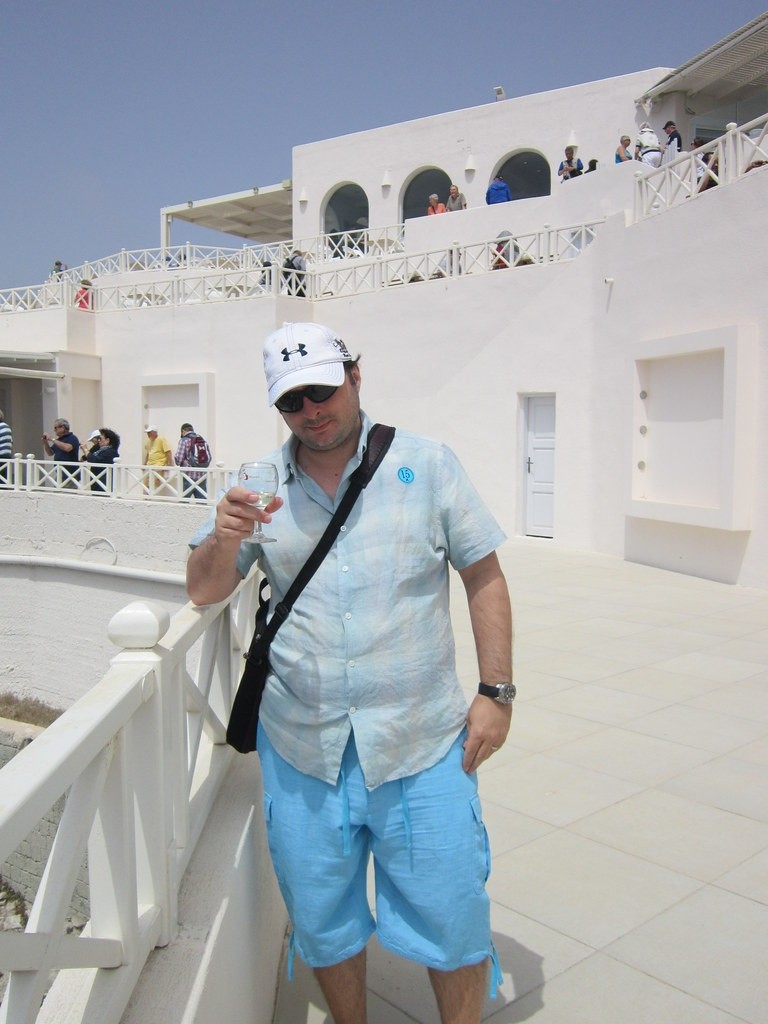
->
[208,284,251,299]
[122,293,162,307]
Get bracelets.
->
[51,439,55,442]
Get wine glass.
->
[238,463,279,544]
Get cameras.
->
[43,435,46,439]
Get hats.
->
[180,423,192,438]
[262,322,352,407]
[88,429,101,442]
[662,121,675,129]
[144,425,158,433]
[493,175,504,180]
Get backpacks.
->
[185,434,211,468]
[283,256,297,280]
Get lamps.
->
[187,200,193,208]
[466,153,477,172]
[380,170,391,186]
[567,131,578,149]
[252,186,258,194]
[299,186,308,201]
[494,86,505,101]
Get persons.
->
[427,194,446,216]
[166,256,175,267]
[281,249,306,297]
[51,260,63,282]
[41,418,81,489]
[328,229,369,259]
[79,428,120,497]
[141,426,174,500]
[0,410,13,484]
[688,132,767,193]
[177,254,187,267]
[486,175,512,205]
[615,120,682,168]
[259,261,283,288]
[190,321,517,1024]
[558,146,584,183]
[445,185,467,212]
[174,422,212,505]
[74,279,93,313]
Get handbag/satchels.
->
[225,598,272,754]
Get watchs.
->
[478,682,517,705]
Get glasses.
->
[54,425,63,429]
[274,384,338,413]
[691,143,695,145]
